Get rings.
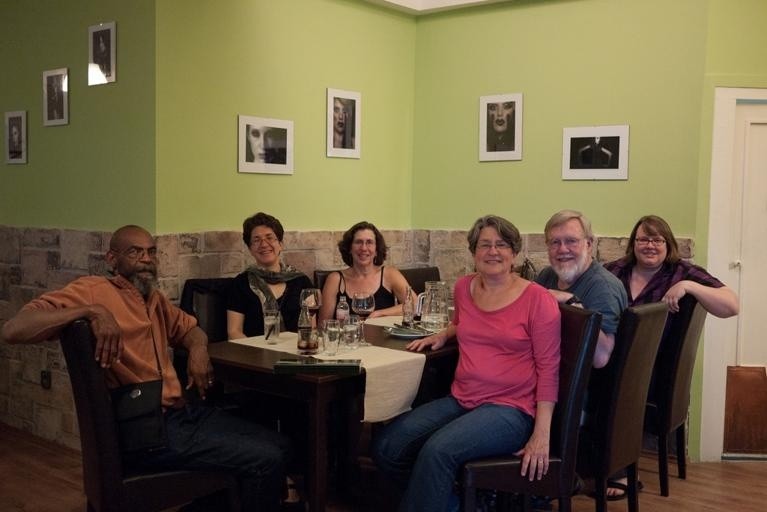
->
[208,380,215,384]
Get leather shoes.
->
[282,484,301,512]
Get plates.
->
[388,329,428,340]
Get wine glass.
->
[350,291,374,347]
[298,289,322,355]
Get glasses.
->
[476,241,509,248]
[635,237,667,246]
[545,237,582,247]
[251,234,278,245]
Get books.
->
[272,357,361,375]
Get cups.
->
[322,318,340,354]
[342,324,358,349]
[262,310,280,345]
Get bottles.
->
[335,296,349,347]
[297,299,311,354]
[401,286,413,329]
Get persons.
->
[487,102,514,150]
[224,212,315,341]
[10,125,21,157]
[316,220,420,335]
[333,98,355,149]
[2,224,314,510]
[94,32,110,72]
[531,209,630,460]
[374,213,564,511]
[246,125,280,164]
[600,214,741,502]
[576,137,613,169]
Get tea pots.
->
[416,281,450,332]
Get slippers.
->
[605,481,644,501]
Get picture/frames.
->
[1,109,28,165]
[477,93,526,161]
[86,19,118,88]
[236,113,296,174]
[39,67,70,127]
[328,88,361,160]
[563,124,631,180]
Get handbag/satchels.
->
[109,380,162,445]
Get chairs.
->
[182,272,255,411]
[461,305,601,511]
[566,300,670,511]
[309,269,339,318]
[396,266,442,321]
[59,314,244,512]
[644,293,710,498]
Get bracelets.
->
[565,295,584,306]
